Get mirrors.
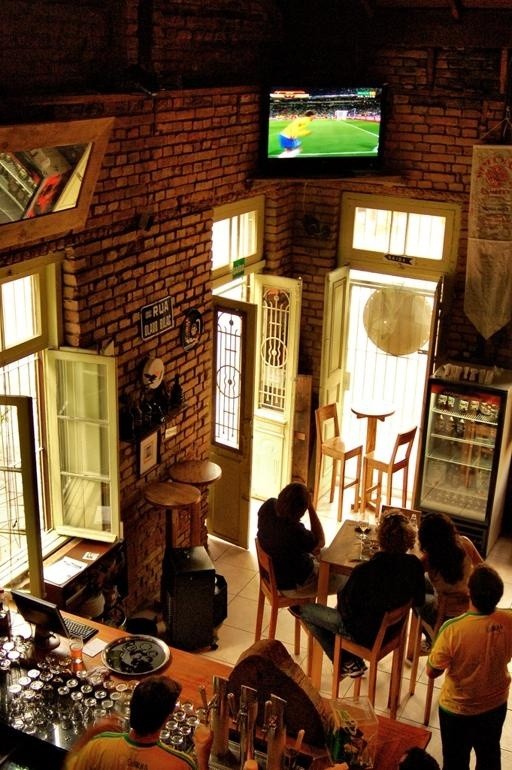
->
[0,117,116,253]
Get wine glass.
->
[359,517,369,539]
[0,635,209,750]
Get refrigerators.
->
[414,377,512,561]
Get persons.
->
[396,747,439,770]
[278,109,317,158]
[298,511,434,673]
[65,677,215,770]
[257,483,349,615]
[427,567,512,770]
[417,514,489,652]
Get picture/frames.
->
[138,427,162,479]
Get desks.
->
[169,461,222,547]
[143,484,201,623]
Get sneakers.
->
[340,661,364,678]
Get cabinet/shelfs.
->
[28,538,129,621]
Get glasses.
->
[384,511,405,522]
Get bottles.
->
[120,374,183,432]
[410,514,417,528]
[424,391,497,512]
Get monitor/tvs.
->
[259,82,384,169]
